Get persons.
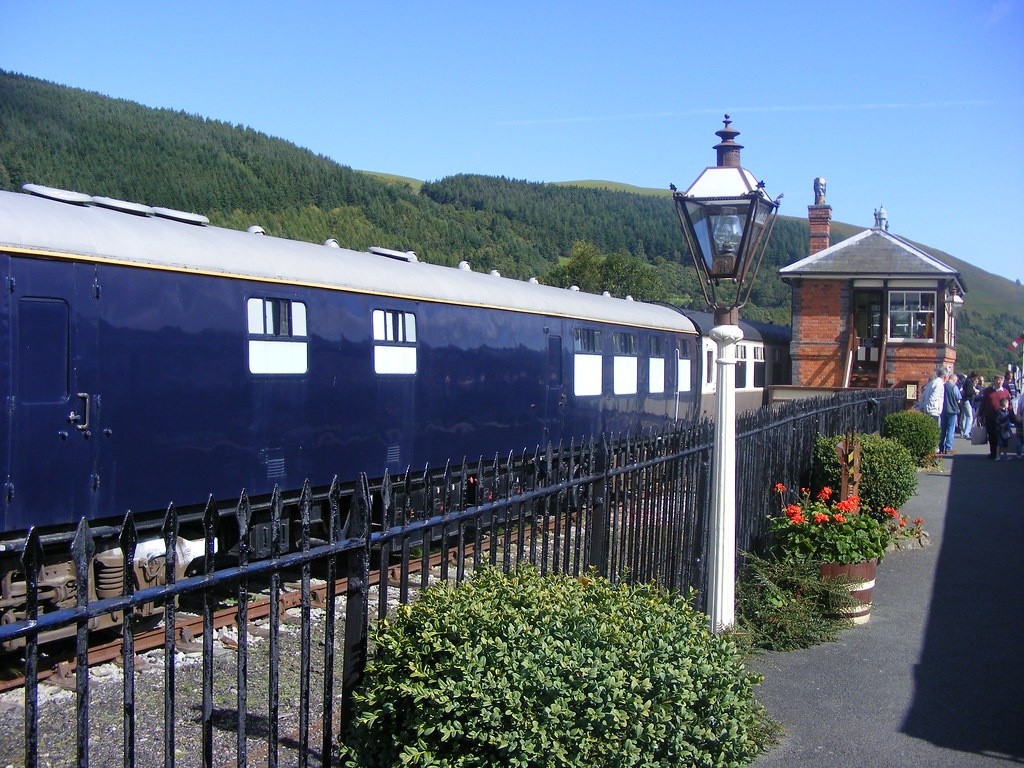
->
[915,368,1024,462]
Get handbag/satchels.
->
[970,417,987,444]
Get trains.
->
[0,182,793,537]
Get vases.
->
[816,560,878,628]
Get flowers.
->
[763,483,929,565]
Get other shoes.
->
[945,450,956,454]
[939,450,945,454]
[1000,454,1012,462]
[964,433,970,439]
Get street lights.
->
[668,114,785,635]
